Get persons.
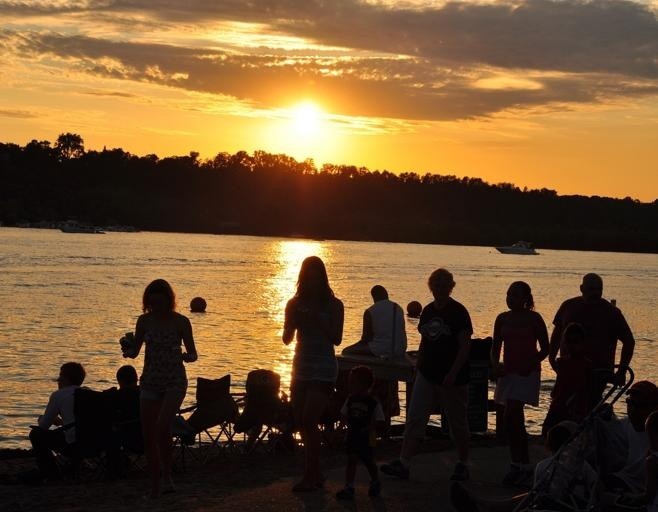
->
[28,361,95,484]
[492,280,550,462]
[282,256,344,492]
[336,365,381,499]
[601,380,657,495]
[542,273,635,442]
[450,411,657,511]
[398,269,473,482]
[113,364,140,417]
[120,279,197,503]
[361,285,407,434]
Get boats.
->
[57,220,106,234]
[496,241,540,255]
